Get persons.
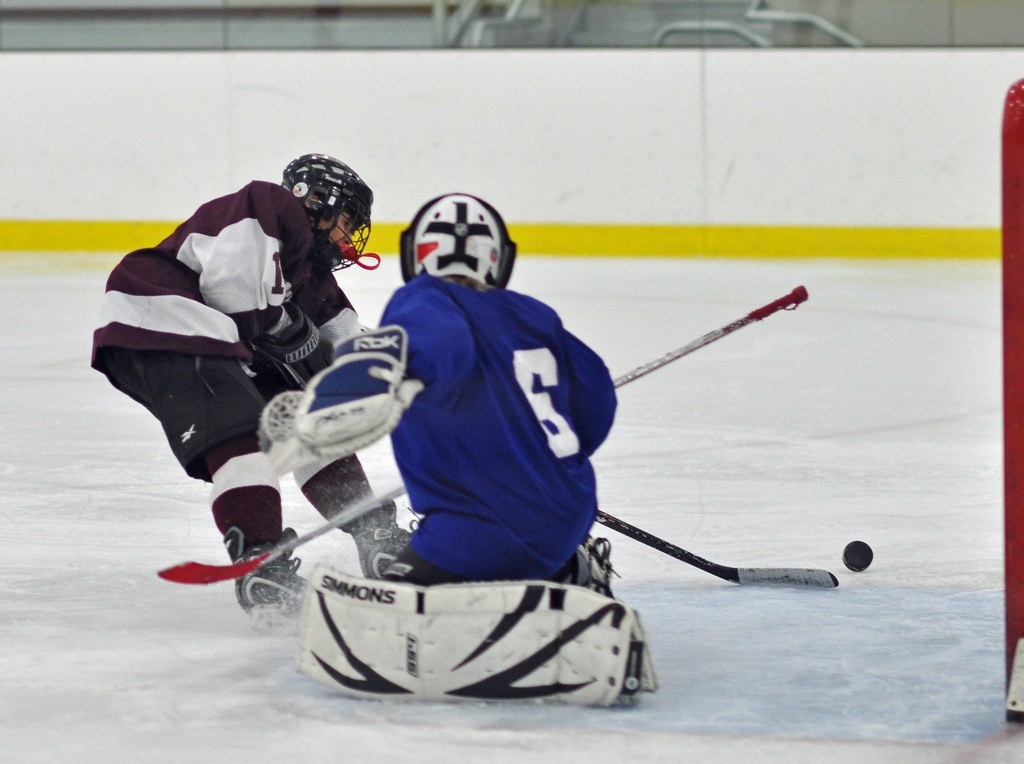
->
[90,153,413,618]
[295,192,660,709]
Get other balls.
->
[842,540,874,572]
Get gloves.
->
[254,300,333,381]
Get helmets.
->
[399,192,516,286]
[283,153,373,270]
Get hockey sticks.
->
[593,506,841,590]
[154,283,812,588]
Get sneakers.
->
[349,503,422,580]
[222,525,309,633]
